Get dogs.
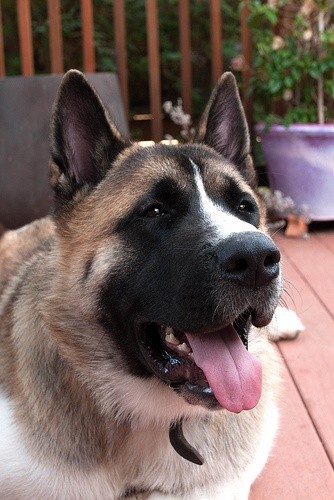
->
[0,68,306,500]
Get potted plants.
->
[237,1,334,219]
